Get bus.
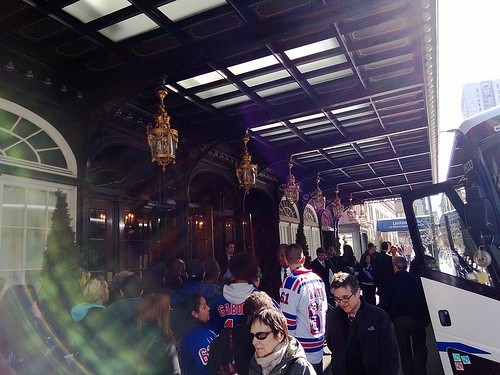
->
[400,105,500,375]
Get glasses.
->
[333,293,354,303]
[250,331,273,341]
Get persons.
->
[248,308,319,375]
[325,272,404,375]
[1,241,441,375]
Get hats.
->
[187,260,204,277]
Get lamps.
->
[232,127,258,195]
[144,78,179,173]
[124,209,138,238]
[331,188,343,220]
[279,160,299,203]
[344,196,357,223]
[310,177,324,211]
